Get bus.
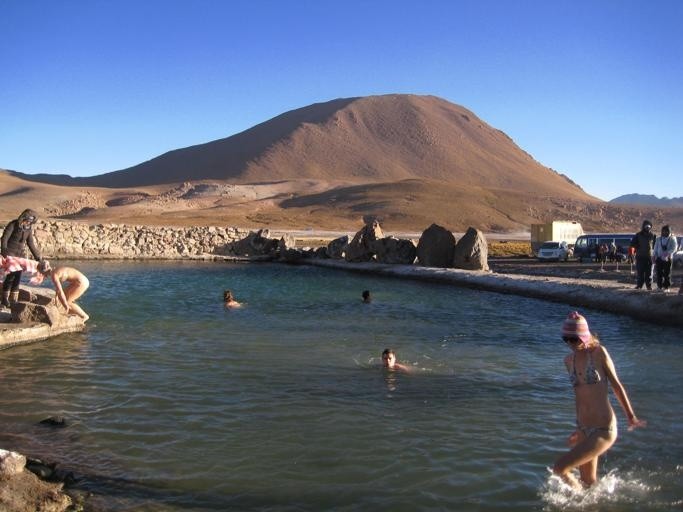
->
[573,234,636,262]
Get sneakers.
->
[635,284,670,293]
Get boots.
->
[1,290,19,307]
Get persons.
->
[380,348,414,372]
[36,260,91,323]
[0,208,45,308]
[578,220,682,294]
[361,289,373,304]
[222,290,241,309]
[550,311,648,488]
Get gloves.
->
[1,248,9,258]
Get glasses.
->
[562,336,579,343]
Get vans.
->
[538,241,568,262]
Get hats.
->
[561,311,591,348]
[26,215,34,224]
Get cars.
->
[673,236,683,269]
